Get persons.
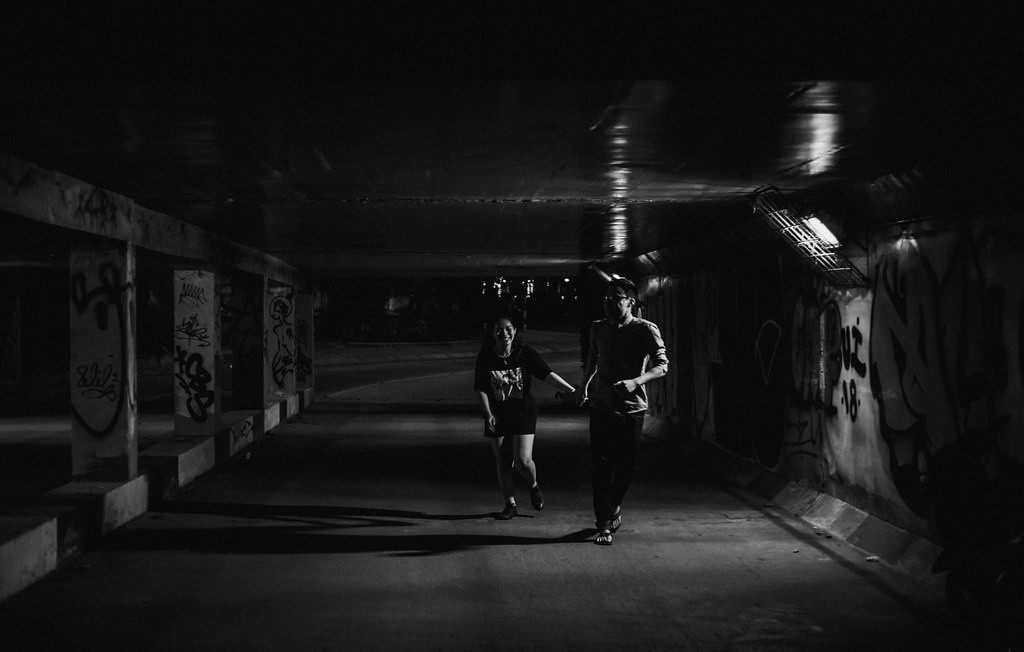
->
[474,313,589,520]
[572,278,671,545]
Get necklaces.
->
[498,356,513,366]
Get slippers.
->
[595,532,613,546]
[610,504,621,531]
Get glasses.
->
[601,296,628,305]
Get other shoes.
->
[528,484,545,511]
[493,502,518,520]
